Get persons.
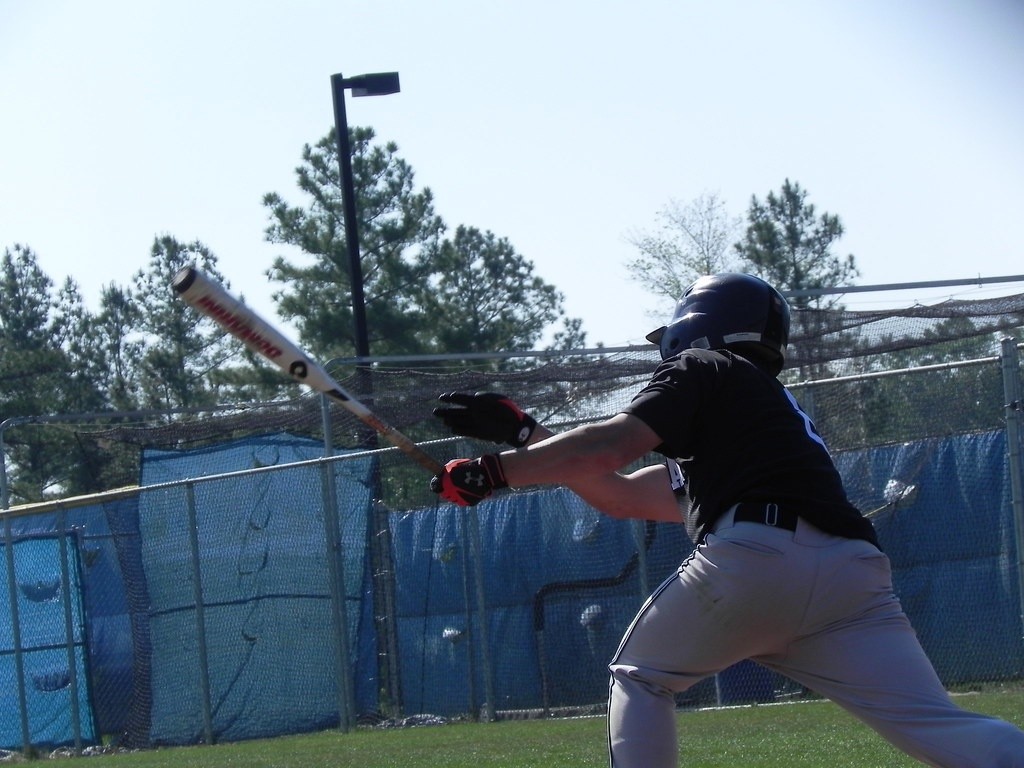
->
[427,273,1024,768]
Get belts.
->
[736,501,796,531]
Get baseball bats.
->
[170,262,446,476]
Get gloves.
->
[430,451,508,508]
[433,391,537,449]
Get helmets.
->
[646,273,790,377]
